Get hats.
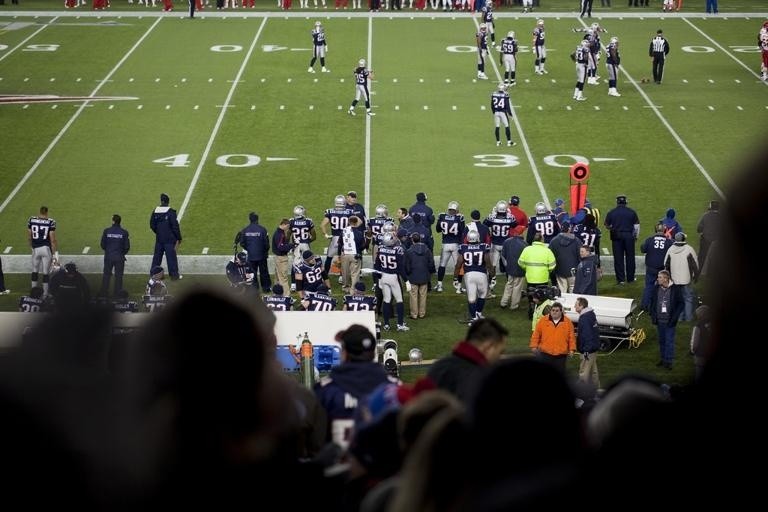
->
[249,212,258,221]
[555,199,565,205]
[417,191,427,200]
[616,196,626,204]
[335,324,376,351]
[316,283,330,292]
[355,283,366,290]
[150,267,163,278]
[667,208,675,218]
[479,74,488,79]
[338,277,343,284]
[161,193,168,202]
[237,252,246,262]
[708,200,721,210]
[657,30,662,34]
[675,233,687,241]
[273,283,283,294]
[328,289,331,294]
[303,250,314,260]
[511,196,520,205]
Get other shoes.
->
[94,8,97,10]
[64,2,86,8]
[168,9,172,11]
[194,5,254,11]
[107,5,110,8]
[162,8,166,11]
[102,7,105,9]
[369,4,482,12]
[282,5,347,10]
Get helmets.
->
[486,0,492,5]
[448,201,460,210]
[591,23,600,30]
[334,195,346,207]
[383,221,396,231]
[508,31,515,37]
[467,230,480,243]
[383,232,396,247]
[537,19,544,26]
[655,223,666,233]
[498,83,507,92]
[610,36,618,43]
[293,205,306,217]
[581,39,589,46]
[535,202,547,214]
[479,23,486,29]
[376,205,388,217]
[496,200,509,212]
[359,59,366,67]
[315,21,321,27]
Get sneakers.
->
[408,316,418,320]
[528,6,533,11]
[170,275,183,280]
[508,140,516,147]
[592,75,599,80]
[608,91,614,95]
[762,75,768,80]
[434,285,443,292]
[657,80,660,85]
[486,294,496,299]
[580,13,583,18]
[577,96,587,101]
[654,79,657,83]
[656,360,665,367]
[510,305,519,310]
[713,11,718,15]
[610,91,620,96]
[616,282,625,286]
[535,70,542,75]
[588,14,591,17]
[492,42,496,46]
[669,6,673,11]
[348,110,356,116]
[663,7,667,12]
[665,362,673,369]
[496,140,502,148]
[322,66,330,72]
[541,69,548,73]
[366,111,376,116]
[510,82,516,86]
[470,318,477,321]
[455,288,463,293]
[453,281,459,287]
[573,95,578,98]
[500,304,507,309]
[419,316,424,319]
[397,324,409,332]
[588,81,599,85]
[504,82,509,87]
[707,11,711,14]
[307,68,315,73]
[476,312,485,319]
[384,325,391,331]
[523,7,527,12]
[628,279,637,283]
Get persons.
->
[653,144,766,512]
[63,0,720,19]
[648,29,670,86]
[586,23,606,86]
[532,18,549,77]
[1,192,766,512]
[481,1,498,48]
[572,40,591,102]
[490,82,518,148]
[347,58,377,117]
[760,28,768,81]
[475,22,491,81]
[498,31,518,89]
[307,20,332,75]
[757,23,768,81]
[605,37,622,97]
